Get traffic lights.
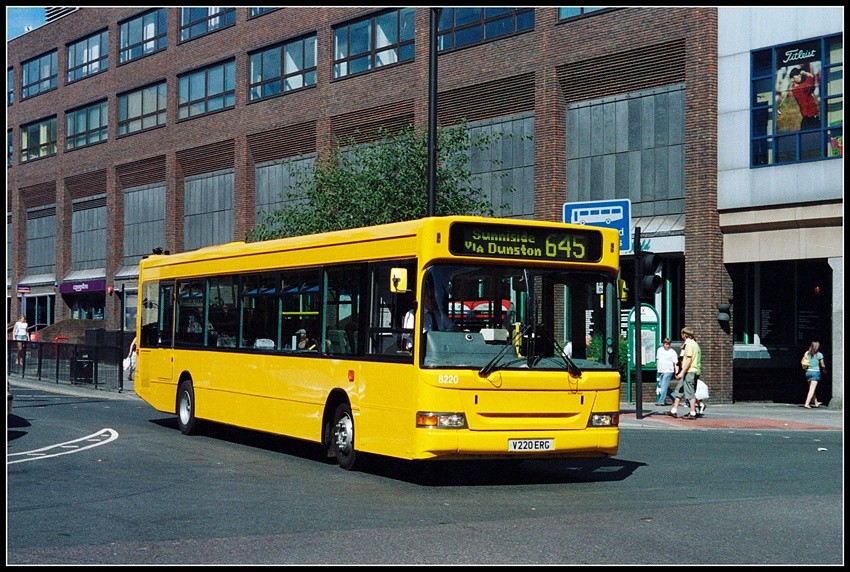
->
[640,251,663,308]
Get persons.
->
[295,322,331,354]
[679,337,708,417]
[561,333,594,360]
[209,298,226,336]
[128,335,138,381]
[663,327,701,421]
[656,337,680,406]
[11,315,30,366]
[400,283,460,353]
[788,64,822,131]
[800,341,827,409]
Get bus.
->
[136,217,620,472]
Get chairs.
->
[174,302,415,357]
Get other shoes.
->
[816,402,822,407]
[682,413,697,419]
[15,358,19,365]
[656,403,665,406]
[699,403,706,414]
[20,358,23,365]
[128,376,133,380]
[664,411,677,417]
[804,405,811,408]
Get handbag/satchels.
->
[694,379,709,399]
[801,351,810,369]
[122,356,131,370]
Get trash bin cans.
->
[70,348,93,383]
